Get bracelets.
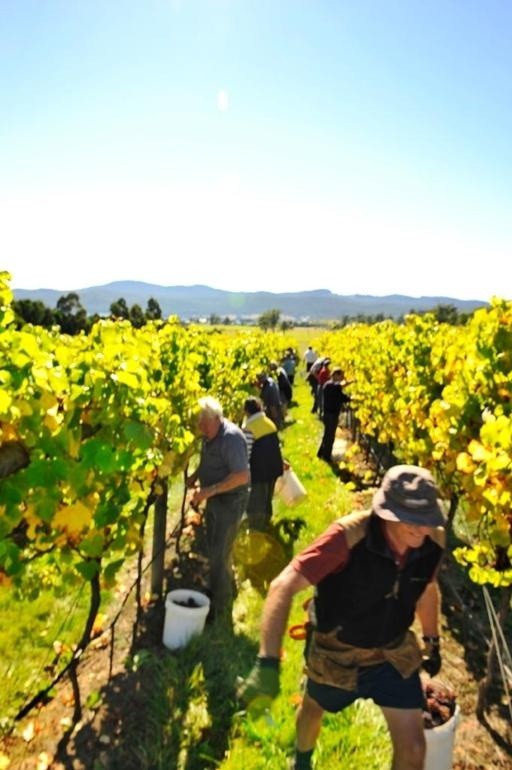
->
[211,483,217,496]
[422,636,440,643]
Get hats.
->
[371,465,444,527]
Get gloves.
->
[422,637,443,677]
[239,654,282,708]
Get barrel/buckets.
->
[332,437,349,463]
[420,670,460,770]
[273,467,308,510]
[162,587,211,650]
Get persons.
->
[184,397,251,625]
[239,397,306,526]
[254,347,360,460]
[236,464,450,770]
